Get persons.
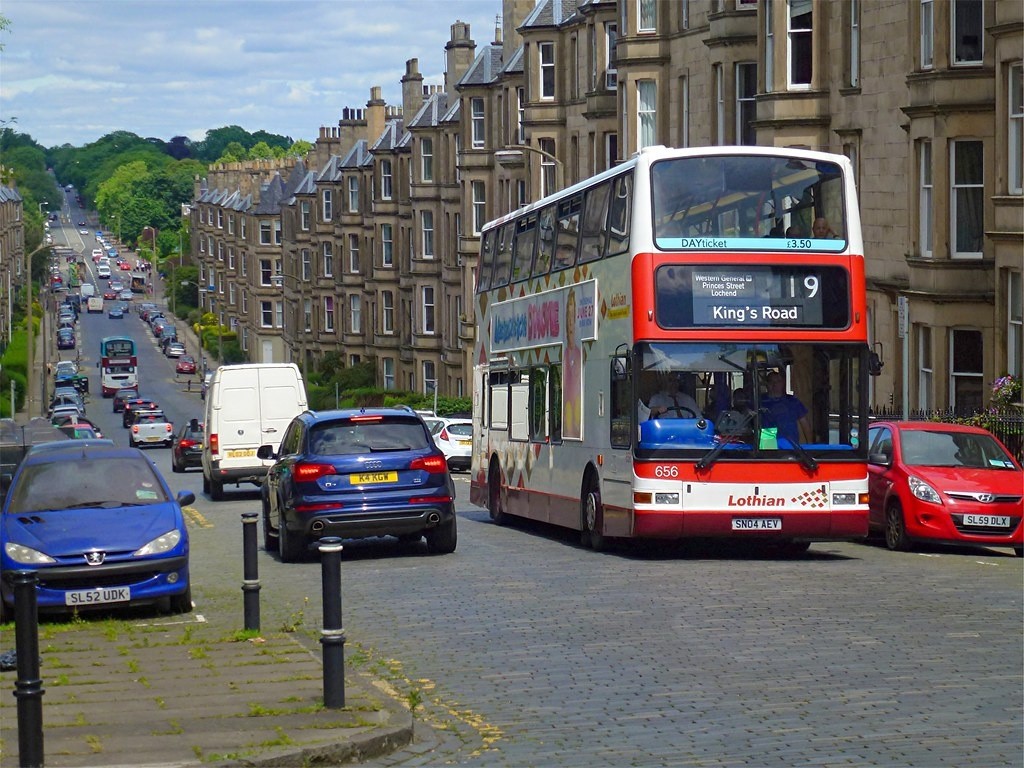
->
[649,373,704,421]
[786,227,801,238]
[564,288,581,439]
[812,217,841,240]
[758,372,813,445]
[733,389,758,437]
[770,227,785,238]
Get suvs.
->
[257,404,457,561]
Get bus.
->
[96,335,139,397]
[469,145,886,560]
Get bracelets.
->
[833,235,840,238]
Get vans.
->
[190,363,309,500]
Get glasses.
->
[668,378,681,383]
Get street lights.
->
[179,279,204,380]
[267,272,309,403]
[39,201,48,216]
[144,226,156,305]
[110,215,121,256]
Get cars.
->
[414,409,472,472]
[111,390,139,413]
[0,446,196,621]
[21,184,199,458]
[123,399,159,429]
[169,420,206,472]
[827,419,1024,558]
[129,410,175,449]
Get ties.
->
[672,396,683,418]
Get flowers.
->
[988,373,1021,405]
